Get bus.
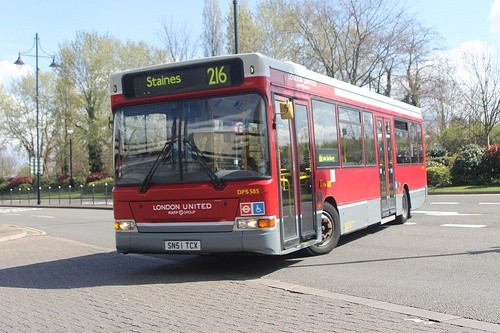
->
[108,53,427,256]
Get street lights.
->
[12,33,60,205]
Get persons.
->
[165,125,192,161]
[202,137,220,163]
[239,150,258,172]
